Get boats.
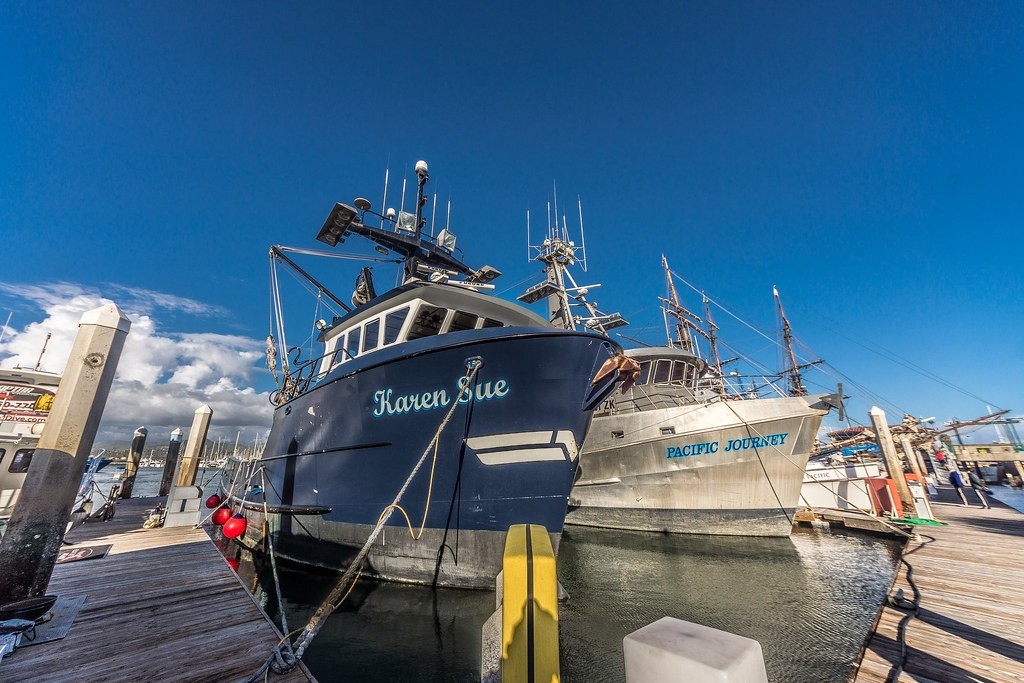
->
[1,332,117,521]
[198,159,1014,605]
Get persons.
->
[966,468,990,510]
[948,470,969,507]
[937,447,948,468]
